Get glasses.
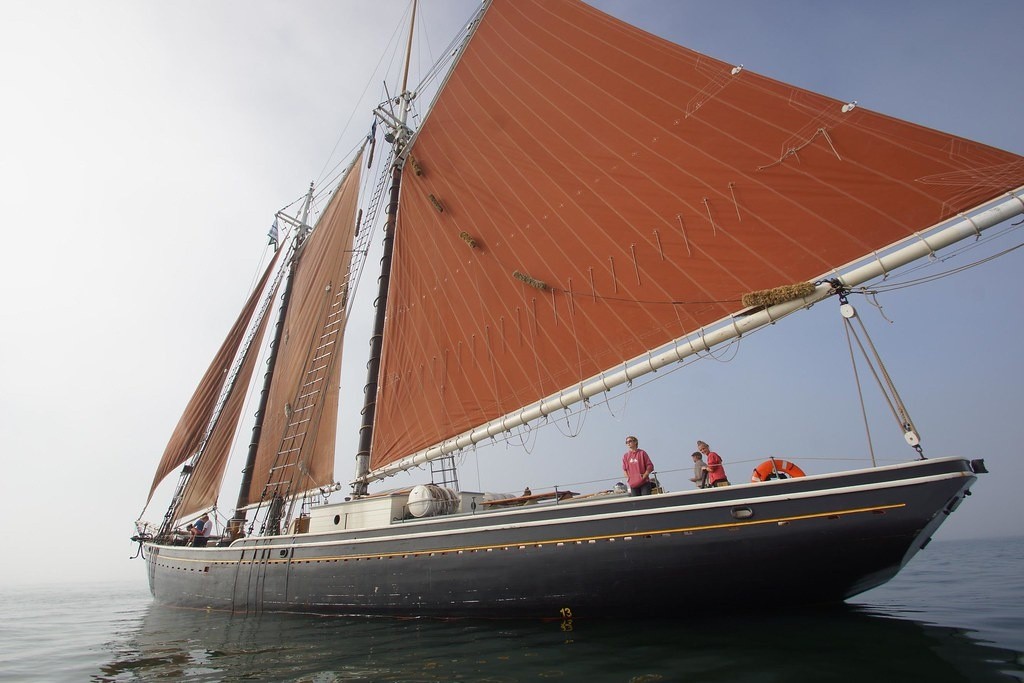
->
[626,440,633,444]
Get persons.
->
[688,452,709,490]
[622,436,655,497]
[697,440,730,488]
[186,513,212,547]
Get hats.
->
[691,452,702,456]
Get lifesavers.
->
[751,460,806,482]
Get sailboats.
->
[129,0,1024,620]
